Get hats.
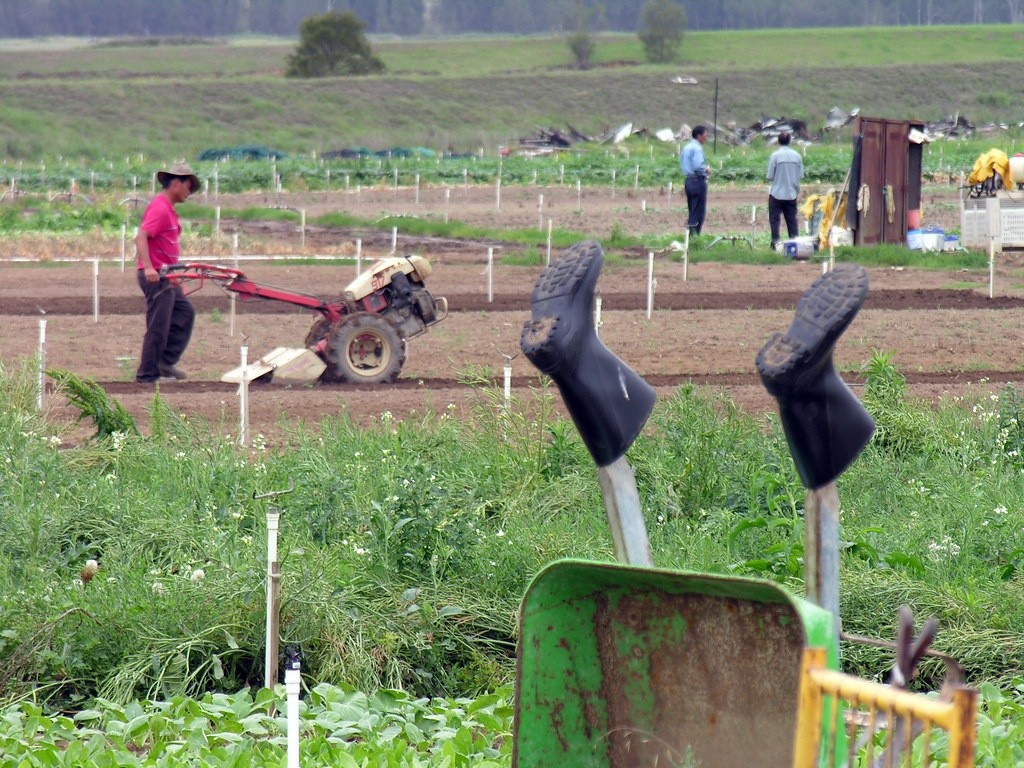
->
[157,163,202,195]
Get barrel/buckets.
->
[905,210,923,250]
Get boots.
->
[755,263,876,490]
[520,238,656,467]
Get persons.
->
[135,163,201,384]
[679,125,711,236]
[766,131,804,247]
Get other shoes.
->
[158,359,185,380]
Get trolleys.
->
[508,456,849,766]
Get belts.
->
[687,175,705,179]
